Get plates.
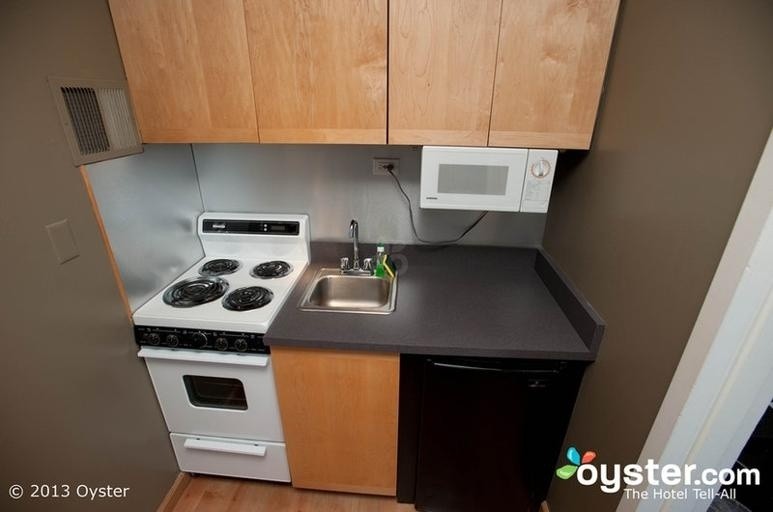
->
[244,0,389,145]
[269,342,400,498]
[388,0,504,147]
[107,0,260,145]
[488,0,623,151]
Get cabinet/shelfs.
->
[374,246,386,277]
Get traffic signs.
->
[373,159,401,176]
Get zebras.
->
[138,353,292,489]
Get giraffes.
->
[131,257,306,351]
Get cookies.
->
[417,144,559,216]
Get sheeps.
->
[349,220,361,269]
